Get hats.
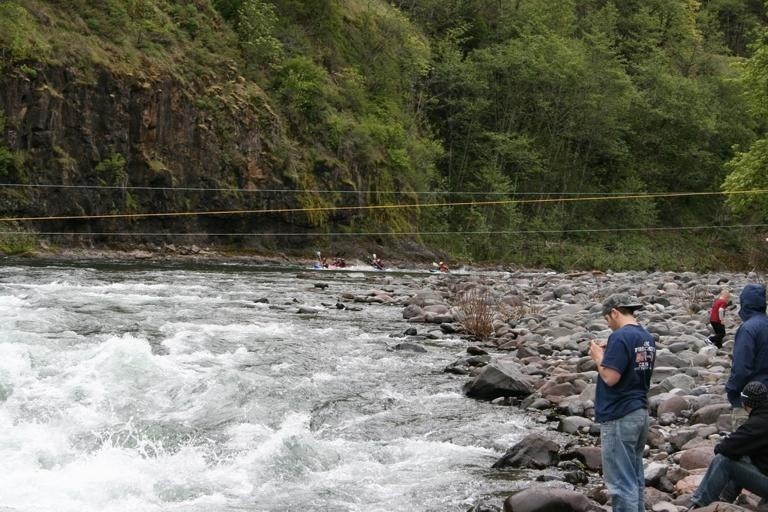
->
[601,293,643,316]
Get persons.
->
[723,281,768,432]
[318,256,329,268]
[438,261,450,272]
[331,256,346,268]
[368,253,383,269]
[685,381,768,511]
[586,292,659,511]
[703,289,730,349]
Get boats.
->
[312,258,450,274]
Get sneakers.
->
[740,381,768,409]
[705,338,712,346]
